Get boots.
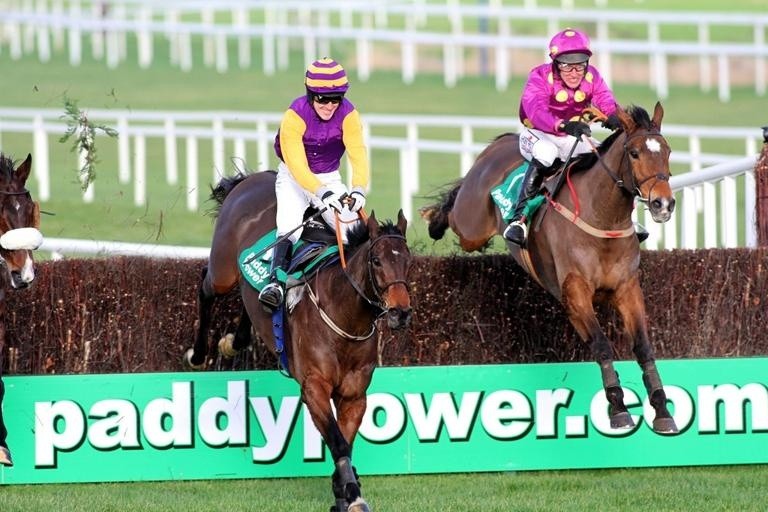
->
[261,236,293,303]
[506,159,545,245]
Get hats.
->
[556,53,589,64]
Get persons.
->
[503,27,650,247]
[258,55,372,309]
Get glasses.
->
[558,64,586,72]
[312,94,341,104]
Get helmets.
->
[550,29,593,62]
[305,58,349,93]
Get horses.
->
[409,100,680,435]
[0,151,44,467]
[180,154,414,512]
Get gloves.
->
[564,120,591,142]
[348,188,366,213]
[318,188,344,214]
[601,116,622,131]
